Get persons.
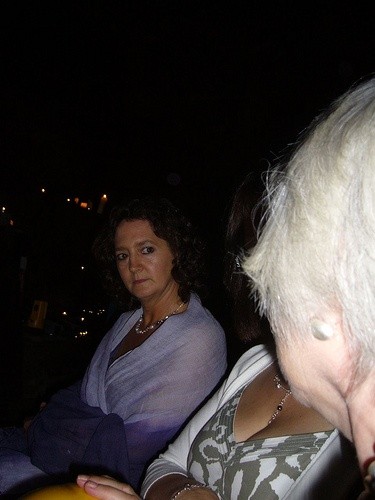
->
[76,77,375,500]
[0,203,228,500]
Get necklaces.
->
[135,301,184,335]
[268,373,293,424]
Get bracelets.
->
[171,482,218,500]
